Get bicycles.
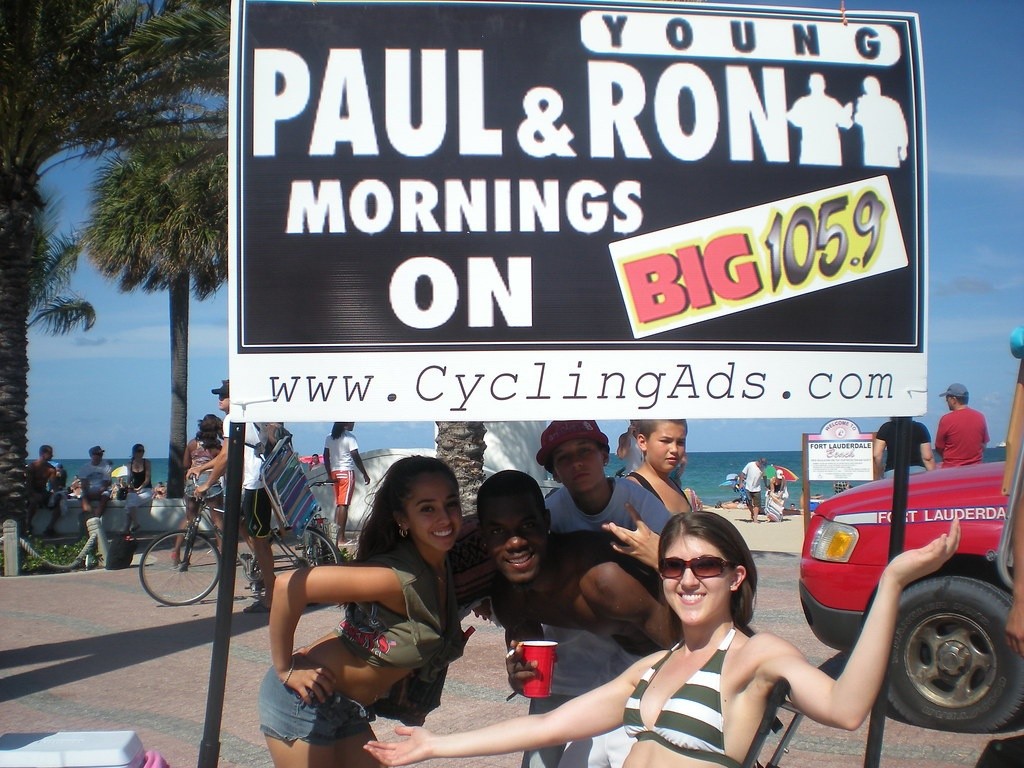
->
[137,473,341,607]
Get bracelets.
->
[281,657,295,684]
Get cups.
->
[519,640,558,698]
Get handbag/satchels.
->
[117,488,128,500]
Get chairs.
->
[751,701,805,767]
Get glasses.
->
[95,452,103,456]
[136,449,145,453]
[658,554,737,578]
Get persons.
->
[257,456,464,767]
[171,414,223,567]
[1003,491,1024,659]
[361,514,960,767]
[476,470,676,768]
[935,384,991,467]
[309,454,320,470]
[618,420,645,472]
[152,481,167,498]
[715,458,799,522]
[26,445,82,538]
[624,420,692,516]
[324,422,370,547]
[81,446,111,519]
[111,484,124,499]
[874,417,935,479]
[535,420,673,574]
[184,379,277,613]
[120,444,152,533]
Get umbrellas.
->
[110,464,127,478]
[765,464,799,482]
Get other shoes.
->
[169,552,182,569]
[120,522,141,533]
[43,528,58,538]
[243,602,269,612]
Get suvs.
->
[796,460,1024,734]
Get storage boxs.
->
[0,728,147,768]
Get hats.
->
[537,416,610,465]
[939,383,968,399]
[89,446,105,457]
[212,381,229,396]
[776,469,785,480]
